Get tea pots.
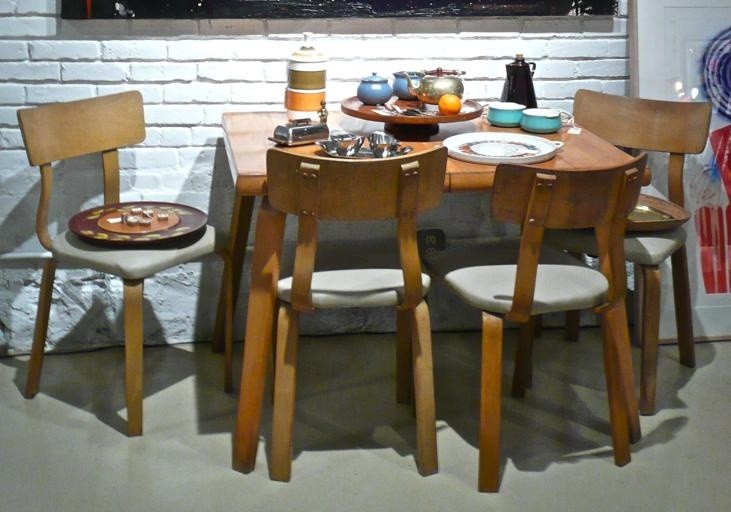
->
[501,54,539,108]
[401,67,467,105]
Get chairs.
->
[17,91,233,436]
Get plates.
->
[316,140,414,160]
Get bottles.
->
[394,72,425,101]
[356,72,392,106]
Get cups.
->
[330,130,401,158]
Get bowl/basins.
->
[488,102,528,128]
[520,108,563,134]
